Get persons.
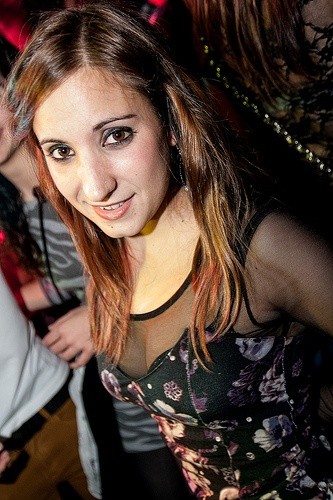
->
[4,3,333,500]
[0,0,333,500]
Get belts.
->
[3,372,73,452]
